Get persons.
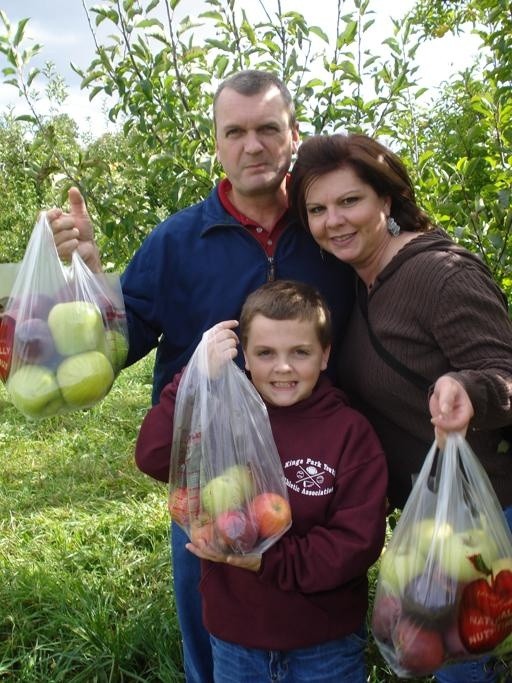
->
[285,136,511,682]
[46,70,370,683]
[134,281,390,683]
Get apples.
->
[2,285,126,414]
[170,458,288,558]
[372,517,512,676]
